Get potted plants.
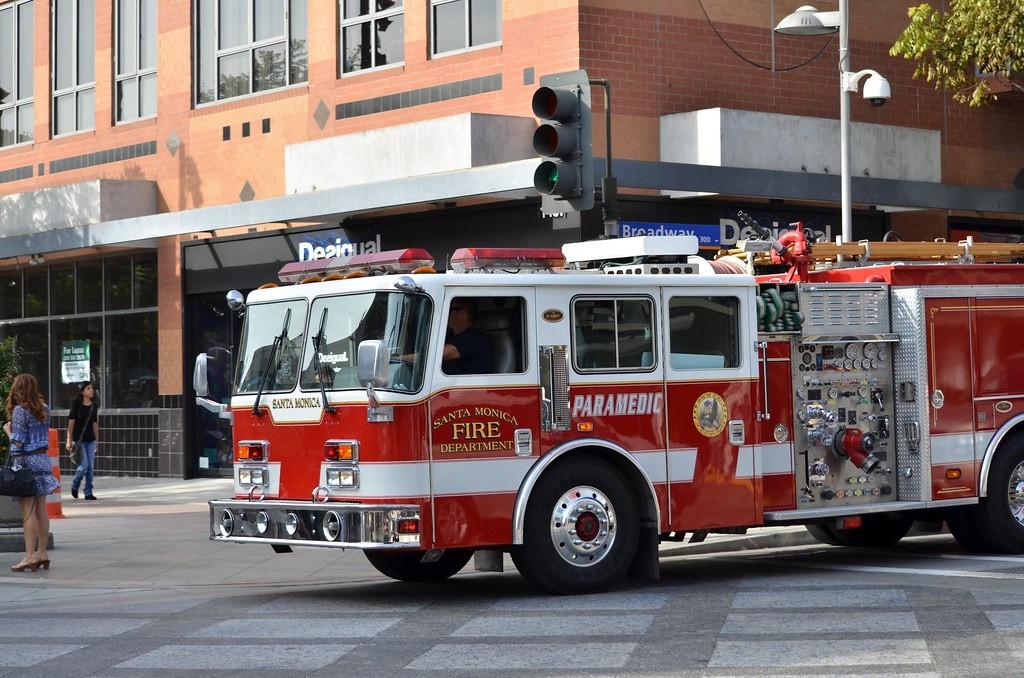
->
[0,336,23,521]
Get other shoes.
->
[85,494,96,500]
[72,488,78,498]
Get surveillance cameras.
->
[862,75,891,107]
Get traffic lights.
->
[531,69,599,213]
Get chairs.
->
[480,307,516,373]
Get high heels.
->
[36,553,50,569]
[11,558,37,572]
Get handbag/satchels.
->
[0,444,36,497]
[71,442,83,464]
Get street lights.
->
[768,0,894,242]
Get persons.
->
[4,374,60,572]
[391,298,492,375]
[315,366,336,382]
[66,381,98,500]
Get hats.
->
[78,381,92,391]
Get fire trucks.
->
[194,235,1024,592]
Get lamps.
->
[29,254,45,265]
